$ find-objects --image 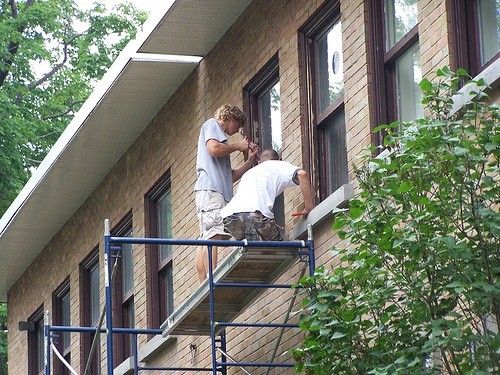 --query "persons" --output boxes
[194,104,259,283]
[220,149,314,241]
